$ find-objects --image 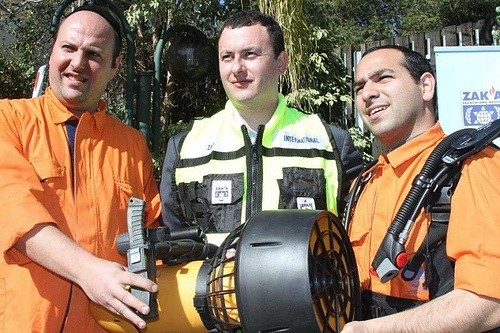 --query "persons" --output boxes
[1,5,162,333]
[342,45,500,333]
[160,11,363,259]
